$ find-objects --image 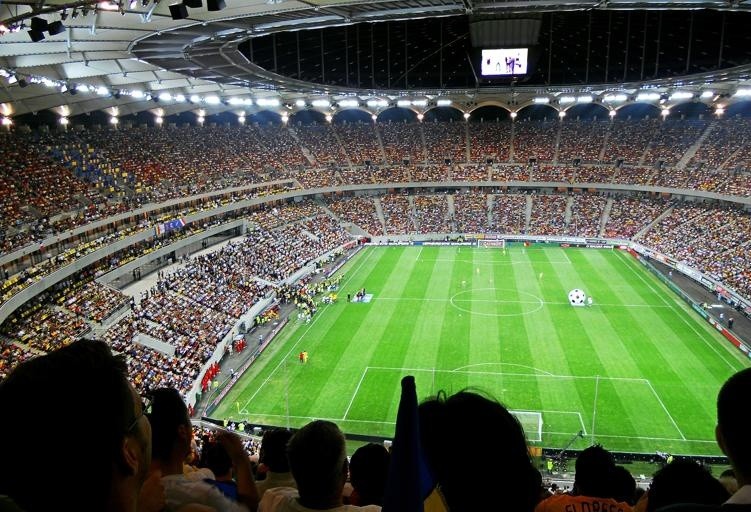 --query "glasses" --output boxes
[128,393,156,432]
